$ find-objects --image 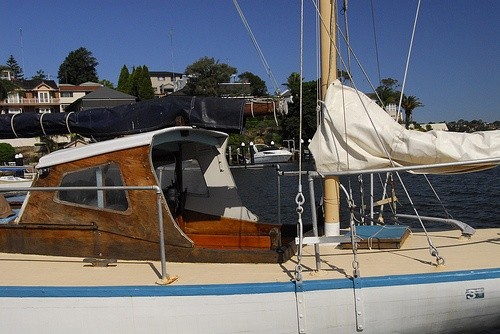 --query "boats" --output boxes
[0,0,500,334]
[0,166,37,187]
[246,144,293,164]
